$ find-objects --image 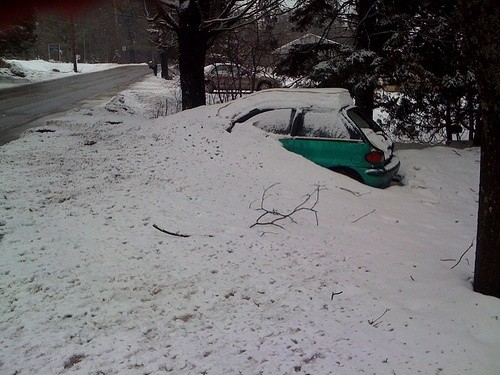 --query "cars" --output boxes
[203,63,282,92]
[214,88,402,191]
